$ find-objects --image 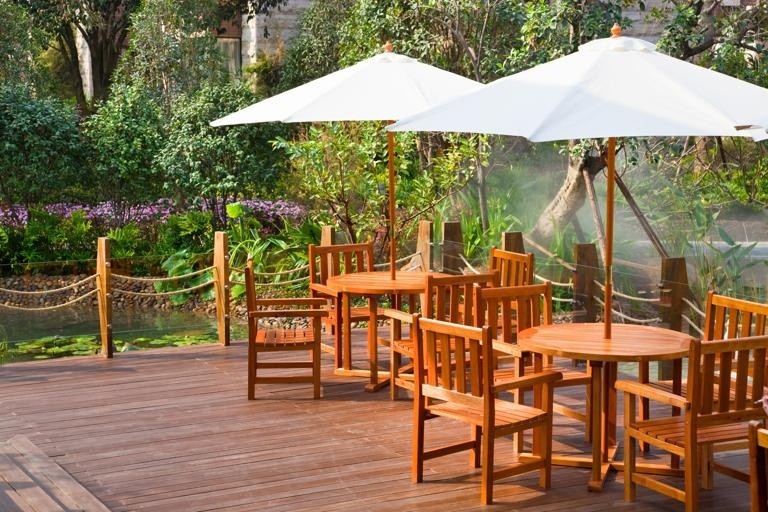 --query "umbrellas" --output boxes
[209,39,486,275]
[385,23,767,336]
